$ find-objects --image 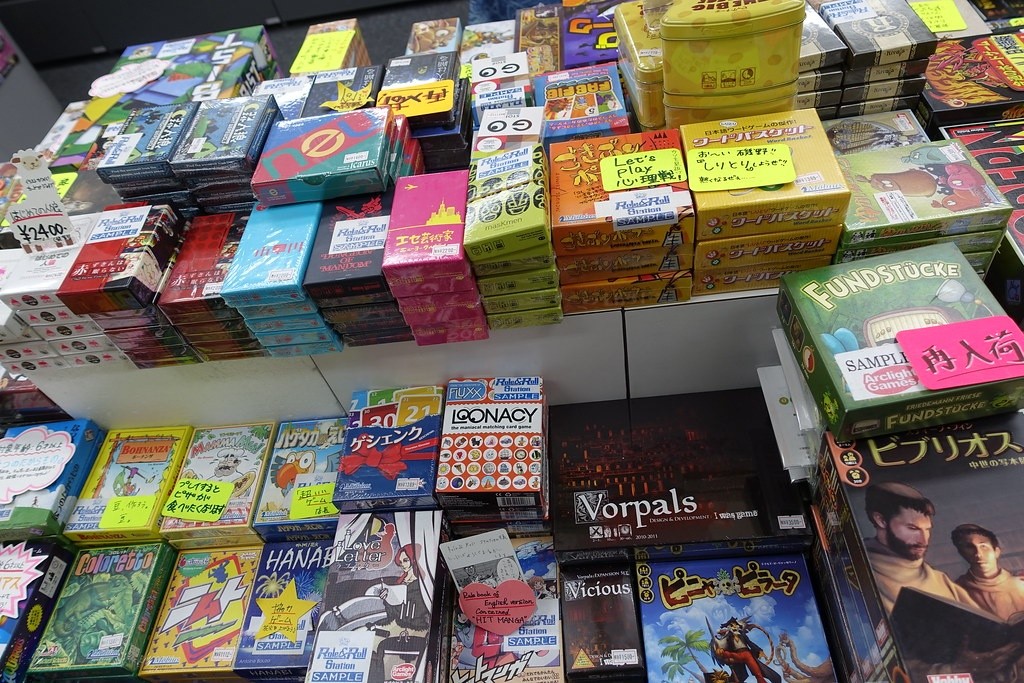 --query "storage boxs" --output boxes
[0,0,1023,683]
